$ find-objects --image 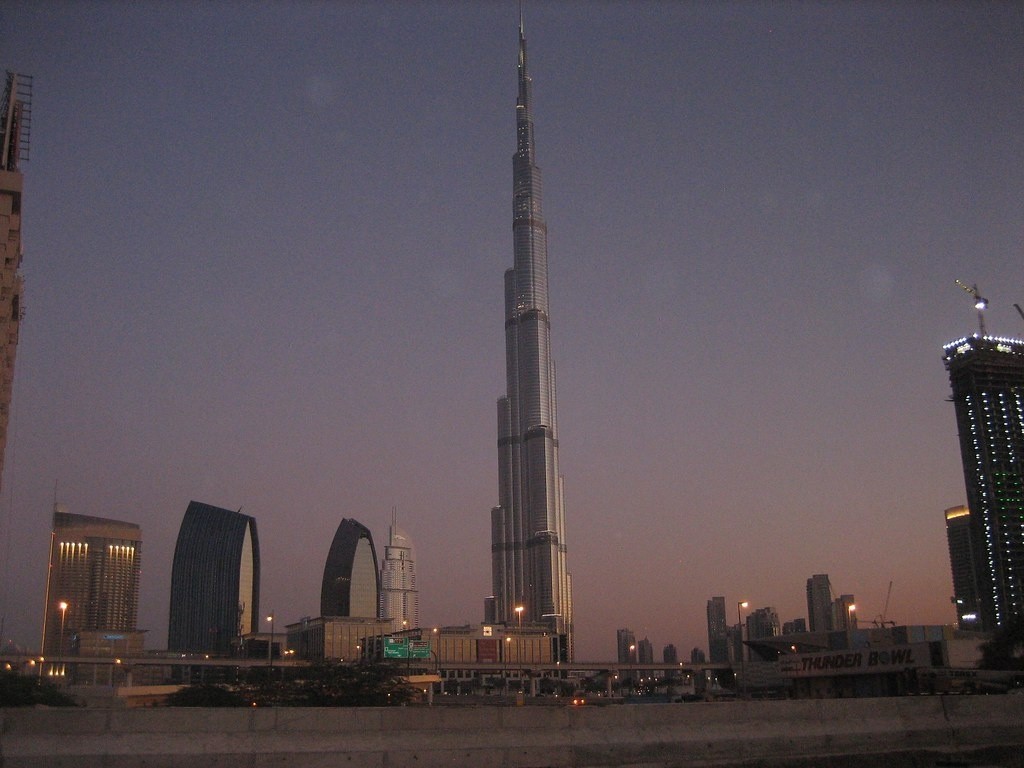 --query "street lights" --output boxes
[60,601,67,655]
[356,646,359,661]
[629,644,634,689]
[507,638,510,664]
[403,618,410,674]
[285,650,294,661]
[267,609,274,684]
[738,601,749,700]
[848,604,855,646]
[962,614,976,631]
[516,607,523,627]
[956,595,963,630]
[429,628,437,642]
